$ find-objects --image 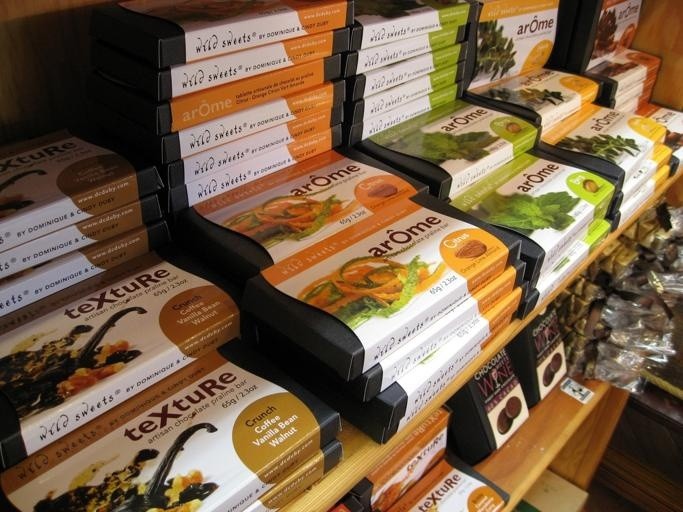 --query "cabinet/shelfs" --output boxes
[0,0,682,512]
